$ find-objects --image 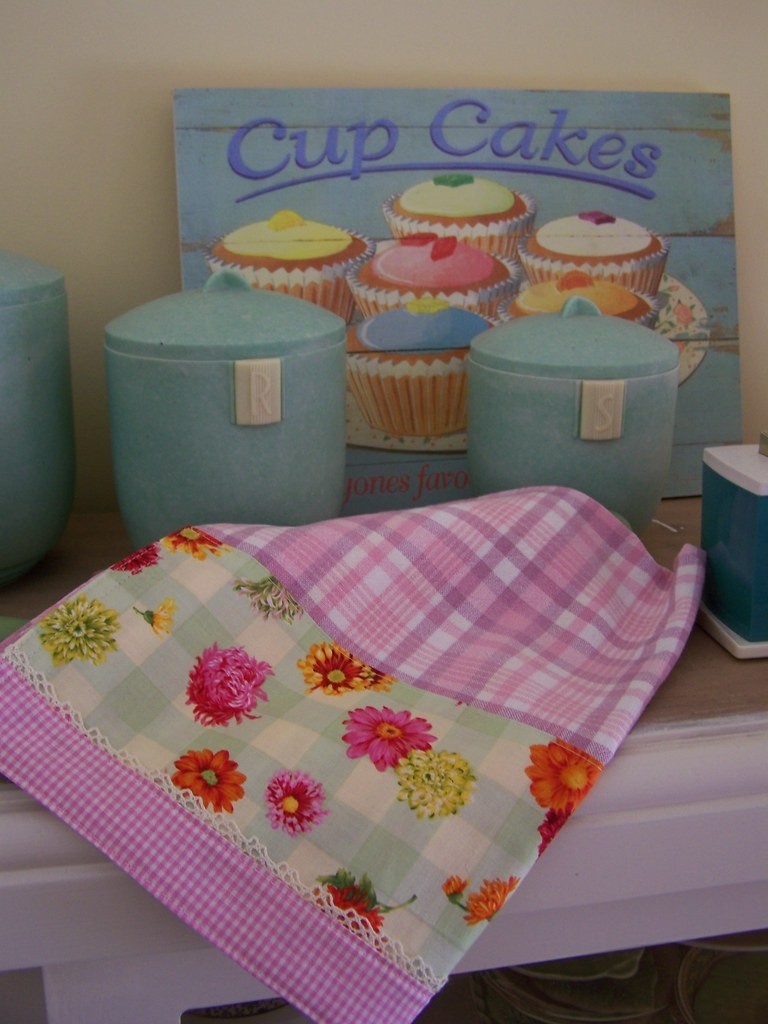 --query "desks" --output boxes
[1,494,767,1024]
